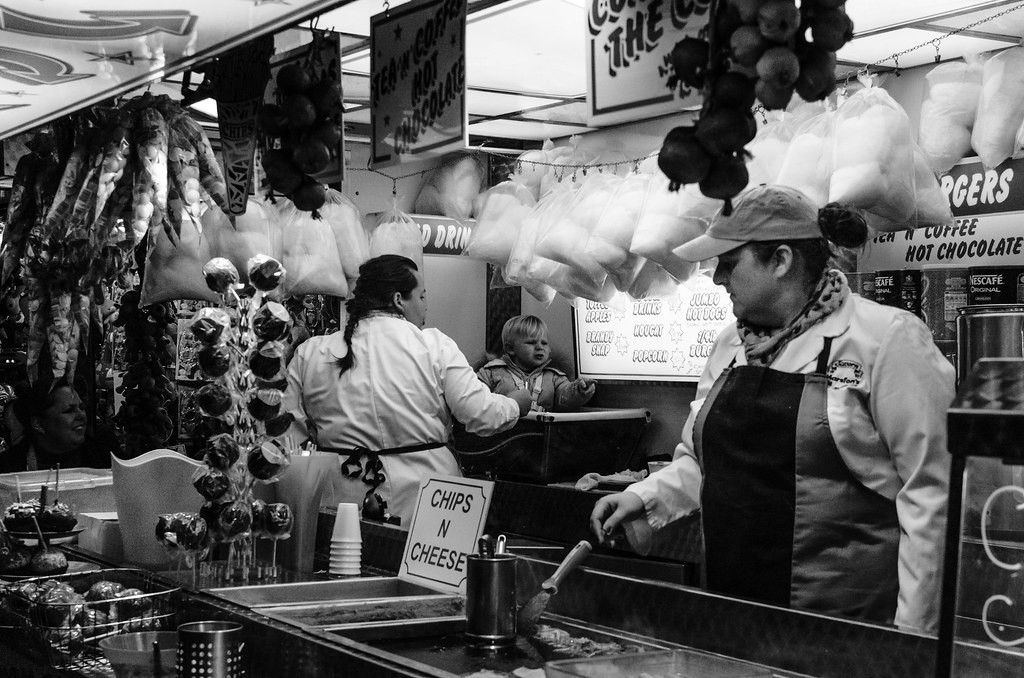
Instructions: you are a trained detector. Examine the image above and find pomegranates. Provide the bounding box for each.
[655,0,855,217]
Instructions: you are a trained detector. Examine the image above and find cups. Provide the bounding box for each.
[328,502,364,575]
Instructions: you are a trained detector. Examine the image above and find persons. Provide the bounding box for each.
[591,182,957,637]
[278,254,534,532]
[0,363,113,473]
[475,314,598,412]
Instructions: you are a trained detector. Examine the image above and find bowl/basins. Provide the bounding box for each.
[100,627,182,677]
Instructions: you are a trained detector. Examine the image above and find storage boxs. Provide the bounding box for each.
[78,511,123,559]
[0,467,118,537]
[452,407,652,486]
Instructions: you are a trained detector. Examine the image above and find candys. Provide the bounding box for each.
[0,460,155,665]
[154,255,296,586]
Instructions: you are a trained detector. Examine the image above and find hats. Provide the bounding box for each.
[672,184,823,263]
